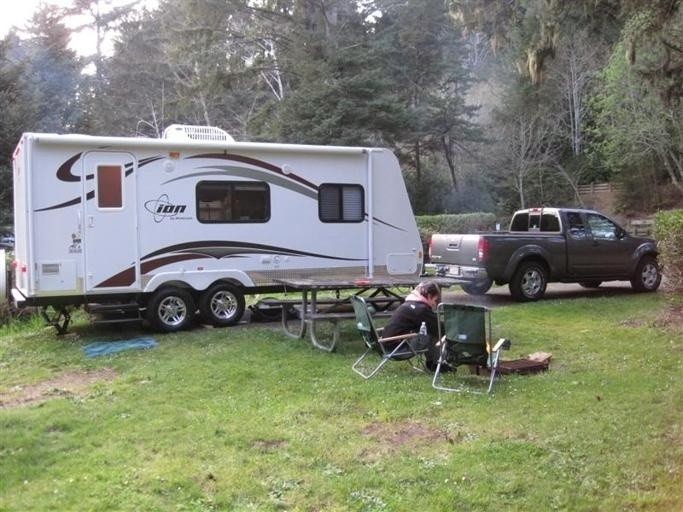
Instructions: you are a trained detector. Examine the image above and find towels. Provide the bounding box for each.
[81,337,158,357]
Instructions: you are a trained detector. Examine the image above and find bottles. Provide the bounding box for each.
[419,321,427,335]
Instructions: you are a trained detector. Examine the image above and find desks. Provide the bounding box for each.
[270,274,472,336]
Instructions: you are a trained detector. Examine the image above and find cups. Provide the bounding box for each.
[495,222,500,231]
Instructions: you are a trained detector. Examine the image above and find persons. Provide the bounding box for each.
[381,281,458,373]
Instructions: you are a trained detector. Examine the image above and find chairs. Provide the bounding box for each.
[429,299,511,399]
[347,291,440,382]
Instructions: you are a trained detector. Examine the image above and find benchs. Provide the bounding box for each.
[301,312,398,355]
[261,295,405,334]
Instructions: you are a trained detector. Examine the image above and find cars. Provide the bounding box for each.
[0,230,14,249]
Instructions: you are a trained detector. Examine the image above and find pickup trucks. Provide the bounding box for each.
[427,208,662,302]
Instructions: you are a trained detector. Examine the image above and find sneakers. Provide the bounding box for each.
[425,360,457,373]
